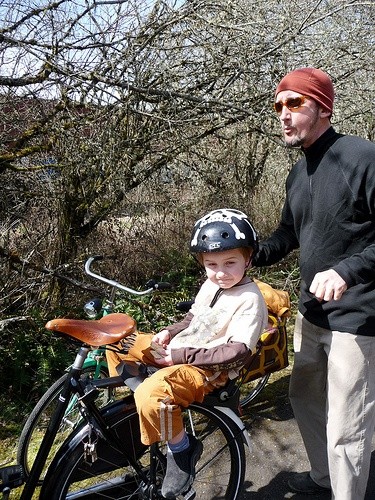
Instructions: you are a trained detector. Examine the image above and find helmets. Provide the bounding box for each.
[190,208,257,253]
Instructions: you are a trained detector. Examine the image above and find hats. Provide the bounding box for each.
[274,68,334,111]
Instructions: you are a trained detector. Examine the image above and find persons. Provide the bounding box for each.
[106,209,268,499]
[240,68,375,500]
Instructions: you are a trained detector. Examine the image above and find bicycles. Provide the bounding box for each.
[17,255,271,487]
[1,313,256,500]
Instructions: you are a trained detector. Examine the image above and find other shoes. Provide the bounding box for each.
[160,434,204,498]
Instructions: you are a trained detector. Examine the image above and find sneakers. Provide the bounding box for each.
[288,473,330,493]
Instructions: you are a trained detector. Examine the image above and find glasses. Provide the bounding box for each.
[275,96,308,113]
